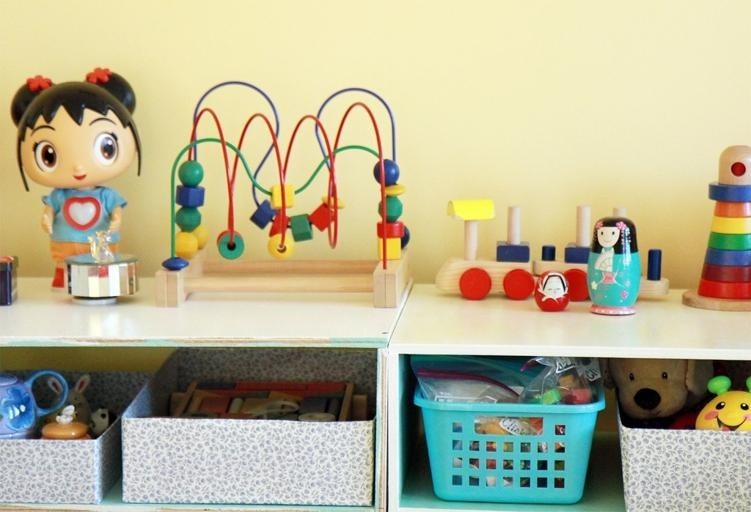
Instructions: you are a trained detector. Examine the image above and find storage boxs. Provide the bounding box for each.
[120,349,374,504]
[0,371,146,504]
[613,395,751,512]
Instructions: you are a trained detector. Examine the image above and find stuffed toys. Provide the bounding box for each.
[467,354,751,488]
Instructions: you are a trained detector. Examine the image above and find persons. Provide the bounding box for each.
[10,68,140,292]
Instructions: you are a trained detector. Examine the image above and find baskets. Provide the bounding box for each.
[413,385,605,506]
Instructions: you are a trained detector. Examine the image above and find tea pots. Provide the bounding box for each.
[0,369,69,438]
